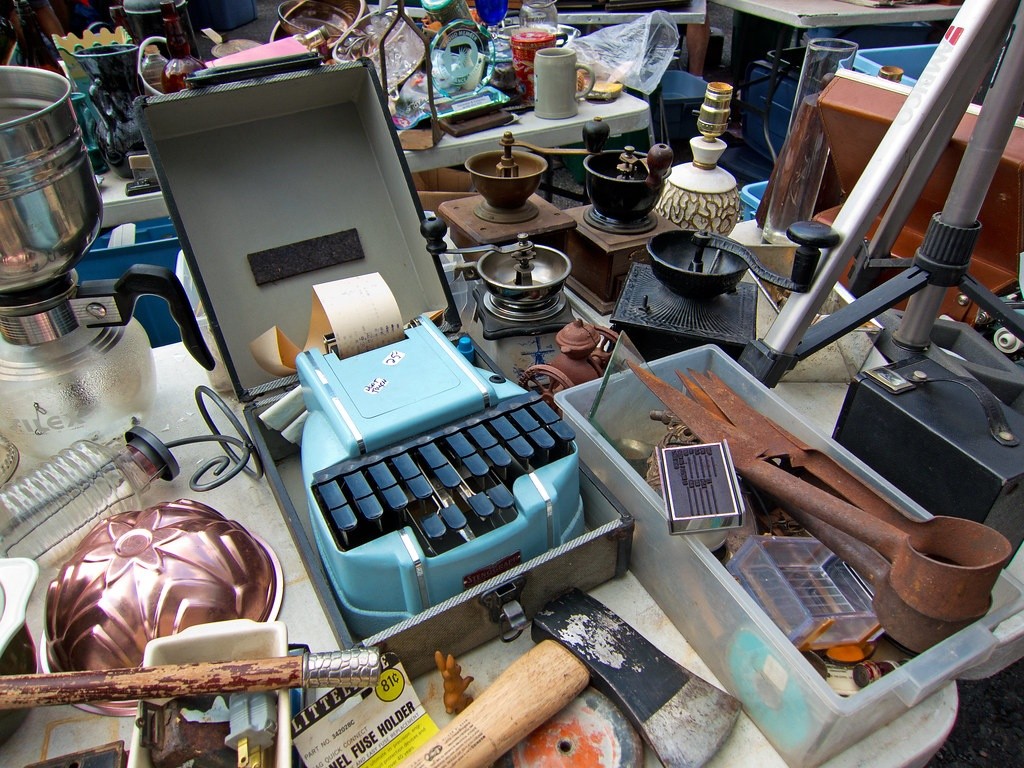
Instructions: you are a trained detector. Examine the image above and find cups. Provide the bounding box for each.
[761,37,859,244]
[513,32,567,106]
[533,48,595,119]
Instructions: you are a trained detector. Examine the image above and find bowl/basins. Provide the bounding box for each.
[331,6,425,91]
[498,24,581,48]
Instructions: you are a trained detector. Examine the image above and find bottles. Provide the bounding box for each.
[71,44,144,179]
[520,0,558,33]
[141,44,169,94]
[292,25,331,52]
[109,6,142,47]
[160,0,208,95]
[0,425,180,598]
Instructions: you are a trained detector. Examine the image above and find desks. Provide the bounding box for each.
[101,84,648,234]
[710,0,966,166]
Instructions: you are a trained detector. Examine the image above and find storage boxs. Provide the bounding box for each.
[129,56,636,674]
[628,66,709,138]
[553,343,1024,766]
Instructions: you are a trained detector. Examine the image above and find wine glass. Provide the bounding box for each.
[475,0,510,52]
